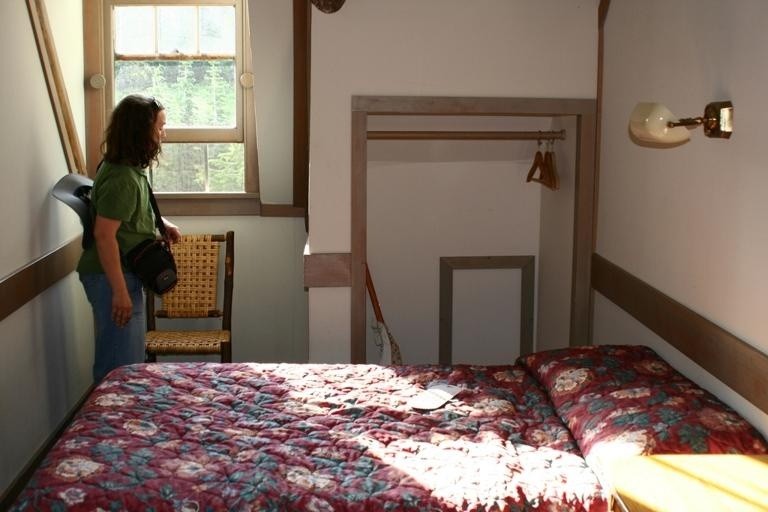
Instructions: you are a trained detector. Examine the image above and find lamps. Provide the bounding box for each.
[630,102,734,144]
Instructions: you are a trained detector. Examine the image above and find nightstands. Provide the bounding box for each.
[612,454,768,512]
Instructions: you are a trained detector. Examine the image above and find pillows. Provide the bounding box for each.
[522,345,768,454]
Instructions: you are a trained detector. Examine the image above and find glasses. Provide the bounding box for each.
[152,100,163,123]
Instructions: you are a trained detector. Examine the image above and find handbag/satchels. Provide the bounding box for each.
[126,239,178,295]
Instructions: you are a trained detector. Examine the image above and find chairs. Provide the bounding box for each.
[143,231,234,362]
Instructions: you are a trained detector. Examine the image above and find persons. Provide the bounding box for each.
[77,94,181,383]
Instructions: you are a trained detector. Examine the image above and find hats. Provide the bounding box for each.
[52,173,95,249]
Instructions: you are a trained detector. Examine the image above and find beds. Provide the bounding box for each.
[25,363,619,512]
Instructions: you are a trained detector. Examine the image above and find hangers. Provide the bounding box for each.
[526,129,560,190]
